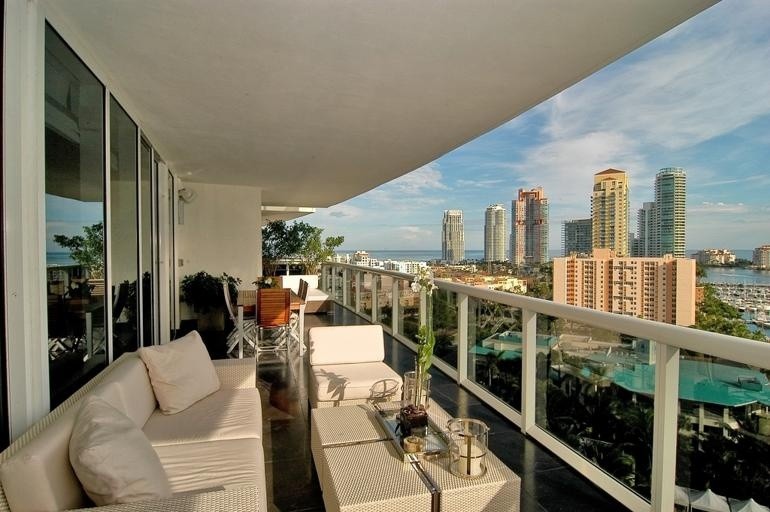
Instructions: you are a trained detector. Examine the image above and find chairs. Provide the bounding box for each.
[271,278,305,345]
[273,281,309,350]
[91,280,131,354]
[222,278,260,354]
[256,288,291,356]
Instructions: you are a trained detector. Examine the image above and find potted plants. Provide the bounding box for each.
[179,270,243,331]
[406,326,435,409]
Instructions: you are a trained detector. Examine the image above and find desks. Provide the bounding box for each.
[236,290,306,359]
[54,289,105,361]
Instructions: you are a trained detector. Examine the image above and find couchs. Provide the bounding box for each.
[281,275,332,314]
[0,353,266,512]
[308,324,405,406]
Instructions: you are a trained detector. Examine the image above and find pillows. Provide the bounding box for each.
[139,330,220,414]
[69,395,173,503]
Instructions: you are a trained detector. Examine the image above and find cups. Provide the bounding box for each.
[400,372,432,411]
[445,418,490,479]
